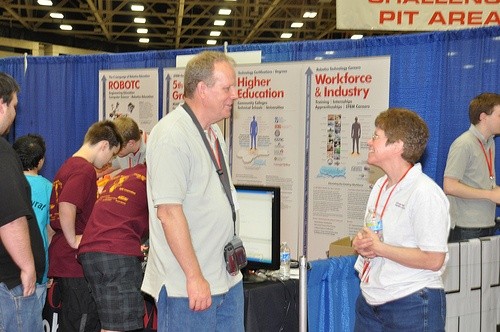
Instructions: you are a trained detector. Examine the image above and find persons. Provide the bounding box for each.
[77,162,149,332]
[443,92,500,243]
[48,119,123,332]
[113,116,149,168]
[16,134,55,312]
[0,71,45,332]
[352,108,451,332]
[141,51,245,332]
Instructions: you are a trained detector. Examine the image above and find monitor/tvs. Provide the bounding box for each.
[234,184,281,276]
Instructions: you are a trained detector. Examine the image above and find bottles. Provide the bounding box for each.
[280,242,291,280]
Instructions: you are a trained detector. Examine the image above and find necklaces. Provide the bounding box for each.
[129,133,147,169]
[477,137,494,180]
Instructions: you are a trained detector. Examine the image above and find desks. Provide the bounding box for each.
[41,279,299,332]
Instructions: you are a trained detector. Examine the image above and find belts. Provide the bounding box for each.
[455,225,497,231]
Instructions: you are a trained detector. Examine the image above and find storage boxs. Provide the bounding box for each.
[329,237,356,257]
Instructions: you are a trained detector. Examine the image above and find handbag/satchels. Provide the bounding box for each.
[47,281,62,312]
[142,300,157,331]
